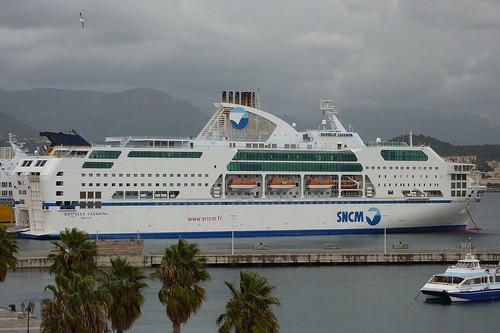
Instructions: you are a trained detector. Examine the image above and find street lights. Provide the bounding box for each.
[383,215,387,253]
[24,301,35,333]
[230,213,236,255]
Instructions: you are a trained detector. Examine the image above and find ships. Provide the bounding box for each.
[11,84,489,240]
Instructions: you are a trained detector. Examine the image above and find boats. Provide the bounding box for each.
[420,238,500,303]
[0,131,28,223]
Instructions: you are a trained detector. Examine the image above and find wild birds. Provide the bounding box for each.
[79,12,85,29]
